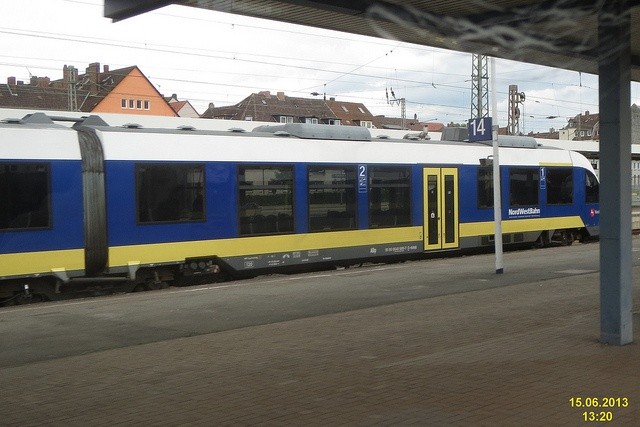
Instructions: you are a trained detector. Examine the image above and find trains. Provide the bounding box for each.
[0,114,601,302]
